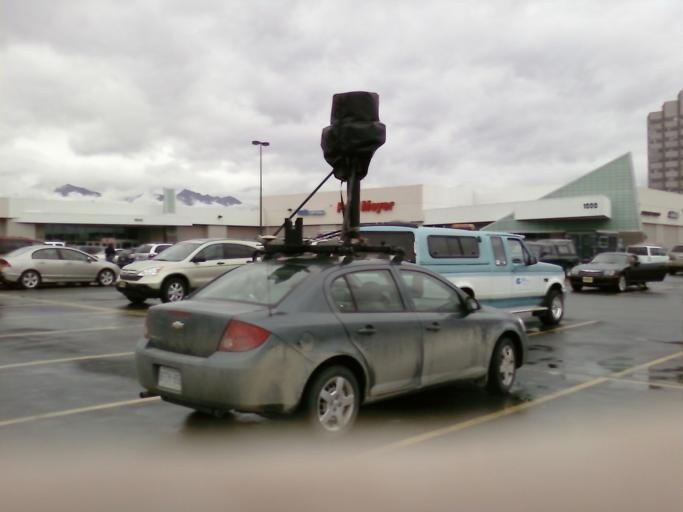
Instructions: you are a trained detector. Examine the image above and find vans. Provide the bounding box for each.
[358,225,564,328]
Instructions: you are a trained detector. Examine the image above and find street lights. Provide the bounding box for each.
[254,140,270,234]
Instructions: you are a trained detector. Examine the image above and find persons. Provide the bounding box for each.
[628,255,649,290]
[104,240,118,264]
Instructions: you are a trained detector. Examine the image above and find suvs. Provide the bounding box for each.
[116,239,263,302]
[535,241,578,272]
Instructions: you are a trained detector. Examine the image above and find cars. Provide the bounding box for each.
[569,243,683,294]
[0,245,119,290]
[138,261,529,439]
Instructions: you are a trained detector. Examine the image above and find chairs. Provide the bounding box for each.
[331,281,392,312]
